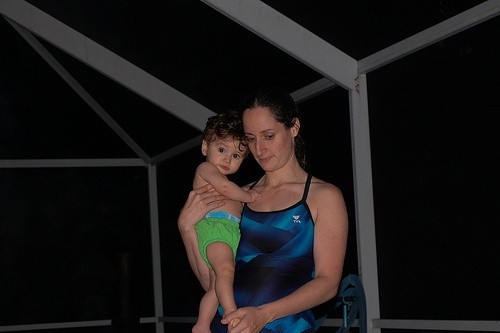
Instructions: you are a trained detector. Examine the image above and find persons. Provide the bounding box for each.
[177,85,349,332]
[192,111,262,332]
